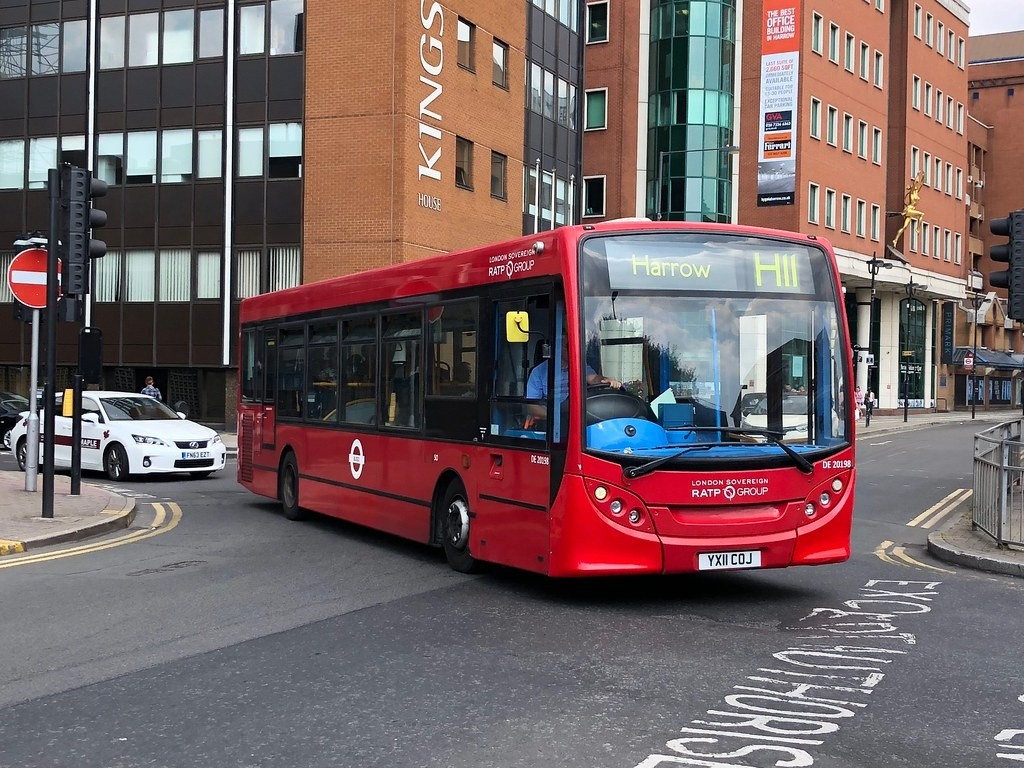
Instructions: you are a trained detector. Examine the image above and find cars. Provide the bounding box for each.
[740,395,839,445]
[0,391,29,449]
[10,391,227,481]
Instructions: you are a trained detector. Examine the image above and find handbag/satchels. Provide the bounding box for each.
[854,408,860,421]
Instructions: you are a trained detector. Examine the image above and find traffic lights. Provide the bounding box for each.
[990,210,1024,325]
[60,164,108,295]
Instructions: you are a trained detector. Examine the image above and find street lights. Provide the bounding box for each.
[904,276,919,422]
[970,292,986,419]
[866,251,884,427]
[14,238,62,491]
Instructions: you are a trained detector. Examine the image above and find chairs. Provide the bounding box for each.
[391,361,406,378]
[435,360,451,385]
[347,353,364,363]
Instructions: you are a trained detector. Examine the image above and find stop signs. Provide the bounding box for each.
[7,247,63,308]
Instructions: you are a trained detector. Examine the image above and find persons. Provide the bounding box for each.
[785,382,876,441]
[141,376,163,418]
[453,361,473,384]
[892,169,928,248]
[306,344,420,409]
[526,331,622,432]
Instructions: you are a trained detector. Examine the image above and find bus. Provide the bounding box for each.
[236,218,853,577]
